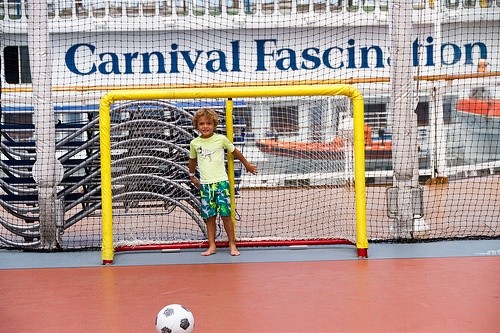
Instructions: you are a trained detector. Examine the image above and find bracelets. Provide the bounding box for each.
[189,172,195,176]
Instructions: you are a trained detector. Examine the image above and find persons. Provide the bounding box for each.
[189,109,258,256]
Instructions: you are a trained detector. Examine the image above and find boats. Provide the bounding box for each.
[254,115,456,175]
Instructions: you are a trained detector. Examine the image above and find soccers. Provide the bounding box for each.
[155,304,194,333]
